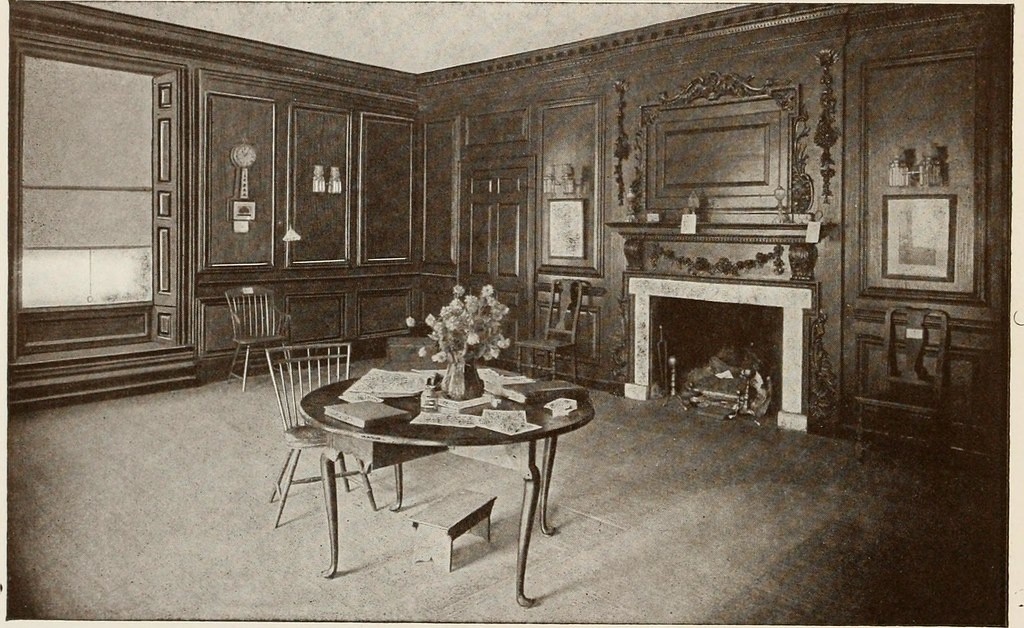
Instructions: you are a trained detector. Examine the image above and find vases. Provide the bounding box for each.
[441,351,485,399]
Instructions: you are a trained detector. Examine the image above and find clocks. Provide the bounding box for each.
[230,143,257,169]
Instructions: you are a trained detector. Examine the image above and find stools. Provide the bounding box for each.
[406,488,499,574]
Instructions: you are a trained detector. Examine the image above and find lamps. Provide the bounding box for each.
[311,165,342,194]
[887,140,941,188]
[542,162,574,196]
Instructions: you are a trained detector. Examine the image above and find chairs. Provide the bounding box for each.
[852,304,952,474]
[262,340,378,527]
[223,287,294,393]
[516,279,583,383]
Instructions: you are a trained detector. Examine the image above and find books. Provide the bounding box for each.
[324,401,411,429]
[502,380,590,404]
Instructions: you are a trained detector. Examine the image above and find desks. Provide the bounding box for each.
[297,366,595,609]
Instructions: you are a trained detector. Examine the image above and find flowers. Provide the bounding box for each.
[405,284,512,363]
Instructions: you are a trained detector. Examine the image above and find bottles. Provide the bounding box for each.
[543,164,555,194]
[313,165,325,193]
[921,141,943,187]
[889,143,908,187]
[420,385,439,409]
[562,164,574,194]
[328,166,342,194]
